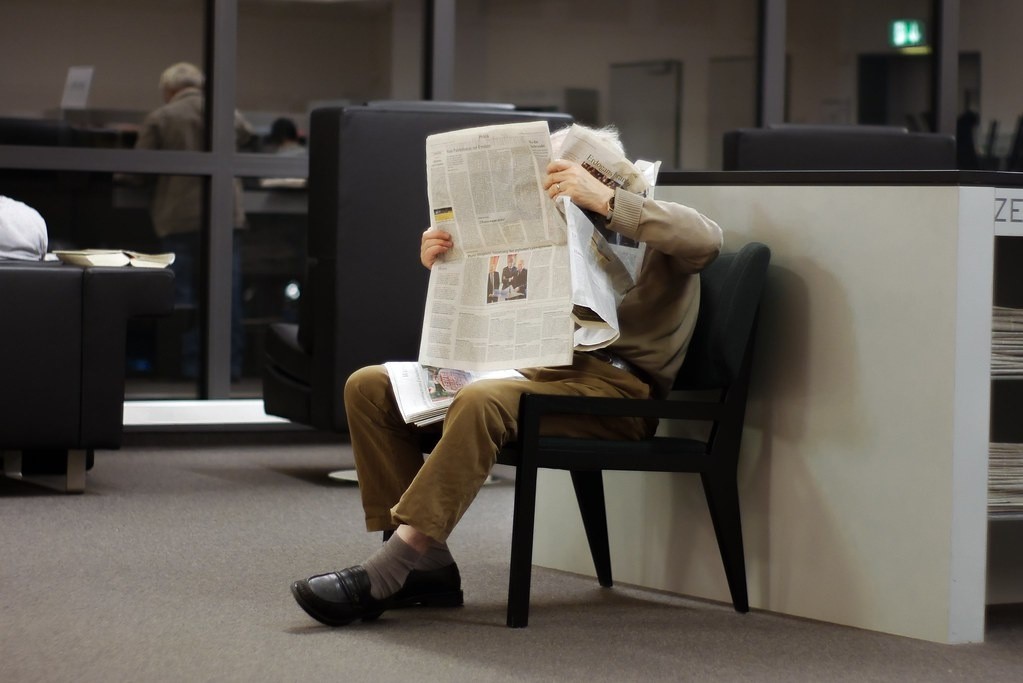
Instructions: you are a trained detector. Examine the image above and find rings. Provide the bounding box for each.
[556,183,561,192]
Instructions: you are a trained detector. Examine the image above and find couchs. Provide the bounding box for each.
[262,108,576,480]
[1,118,142,255]
[0,261,180,492]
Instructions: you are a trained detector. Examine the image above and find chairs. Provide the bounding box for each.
[419,242,770,632]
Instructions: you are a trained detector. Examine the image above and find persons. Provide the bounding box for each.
[501,257,527,301]
[132,64,296,400]
[487,264,500,303]
[582,161,615,189]
[290,122,723,626]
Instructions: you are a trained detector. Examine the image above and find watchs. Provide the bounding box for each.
[607,191,615,219]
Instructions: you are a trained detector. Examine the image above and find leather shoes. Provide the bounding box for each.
[386,562,465,608]
[289,565,423,627]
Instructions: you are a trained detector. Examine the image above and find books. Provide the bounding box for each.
[52,249,175,269]
